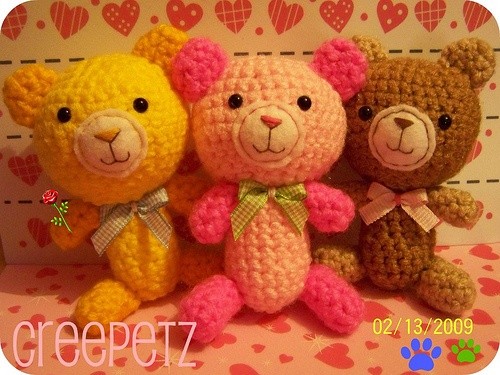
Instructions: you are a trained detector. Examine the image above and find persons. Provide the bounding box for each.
[171,37,369,344]
[3,23,225,337]
[311,35,496,315]
